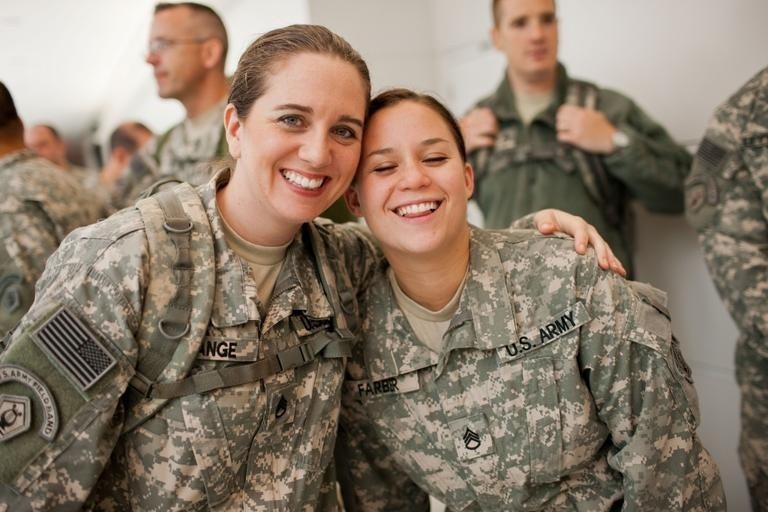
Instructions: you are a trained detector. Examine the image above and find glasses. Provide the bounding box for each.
[147,33,205,53]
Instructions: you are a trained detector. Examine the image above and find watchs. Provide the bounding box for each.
[603,131,631,157]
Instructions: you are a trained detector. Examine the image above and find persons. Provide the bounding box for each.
[0,81,94,340]
[96,2,230,219]
[334,89,726,512]
[0,25,628,512]
[685,67,768,512]
[80,122,154,200]
[457,0,692,282]
[24,125,88,183]
